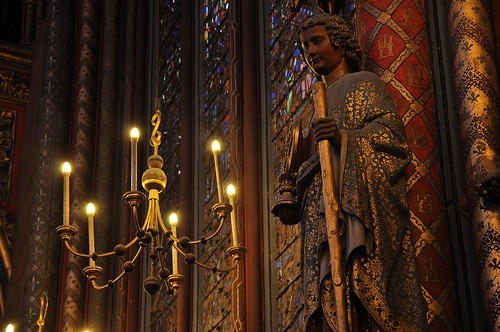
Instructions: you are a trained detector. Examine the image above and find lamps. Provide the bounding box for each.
[57,0,247,305]
